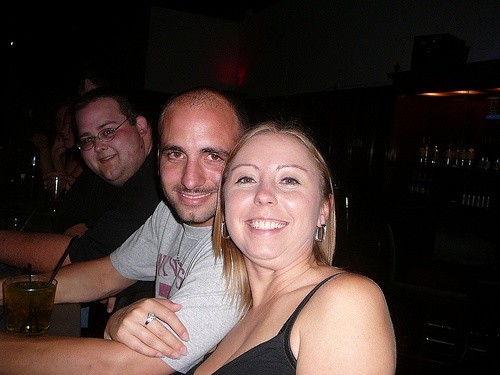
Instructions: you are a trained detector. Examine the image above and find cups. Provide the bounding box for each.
[3,276,58,335]
[46,177,66,198]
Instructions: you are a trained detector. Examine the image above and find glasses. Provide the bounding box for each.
[74,113,138,150]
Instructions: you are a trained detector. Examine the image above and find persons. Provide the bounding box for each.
[2,84,166,341]
[30,98,87,201]
[103,120,397,375]
[1,89,253,375]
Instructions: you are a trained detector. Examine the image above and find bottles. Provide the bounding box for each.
[408,133,500,210]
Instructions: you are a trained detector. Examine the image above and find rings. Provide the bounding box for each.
[144,312,155,326]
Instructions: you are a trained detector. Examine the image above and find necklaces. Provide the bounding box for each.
[176,217,219,267]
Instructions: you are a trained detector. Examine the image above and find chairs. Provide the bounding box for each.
[386,206,485,375]
